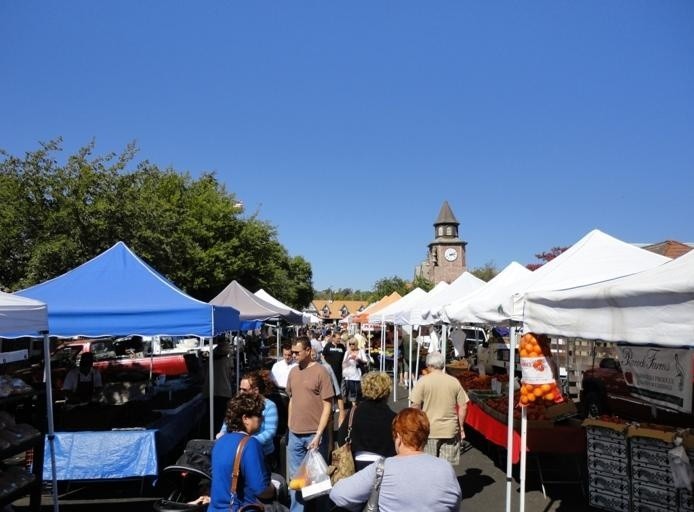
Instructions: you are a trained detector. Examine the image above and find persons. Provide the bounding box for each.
[319,331,346,388]
[328,408,462,512]
[336,372,398,472]
[286,335,335,510]
[216,373,279,463]
[439,331,455,363]
[406,351,469,467]
[310,338,347,433]
[208,391,276,512]
[63,351,101,409]
[403,331,421,380]
[271,342,299,395]
[342,336,368,403]
[203,339,232,424]
[375,323,402,348]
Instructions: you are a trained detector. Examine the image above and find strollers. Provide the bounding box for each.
[151,439,221,512]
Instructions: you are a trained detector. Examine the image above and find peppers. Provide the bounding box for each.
[472,389,496,396]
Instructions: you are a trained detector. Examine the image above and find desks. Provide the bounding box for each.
[456,391,587,499]
[31,391,210,486]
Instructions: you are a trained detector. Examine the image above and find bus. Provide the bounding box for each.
[485,345,575,387]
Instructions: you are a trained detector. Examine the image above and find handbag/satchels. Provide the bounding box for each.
[230,436,268,512]
[331,405,355,486]
[363,458,385,512]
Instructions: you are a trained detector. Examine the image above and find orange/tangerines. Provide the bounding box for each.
[519,333,565,406]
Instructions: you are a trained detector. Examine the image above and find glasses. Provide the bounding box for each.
[239,388,252,392]
[349,343,356,346]
[291,350,301,357]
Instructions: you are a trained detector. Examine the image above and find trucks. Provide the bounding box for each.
[581,342,694,423]
[14,340,204,391]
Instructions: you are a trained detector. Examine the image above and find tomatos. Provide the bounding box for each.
[485,394,521,419]
[640,423,674,434]
[527,402,548,421]
[597,414,626,425]
[494,373,510,383]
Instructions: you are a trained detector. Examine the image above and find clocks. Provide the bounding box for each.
[443,248,458,262]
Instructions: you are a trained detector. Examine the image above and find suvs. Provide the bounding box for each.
[439,324,487,361]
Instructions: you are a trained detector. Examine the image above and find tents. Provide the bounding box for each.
[470,227,673,512]
[368,260,535,407]
[519,248,694,511]
[254,288,323,350]
[1,290,58,512]
[207,280,305,392]
[337,291,403,372]
[15,240,239,442]
